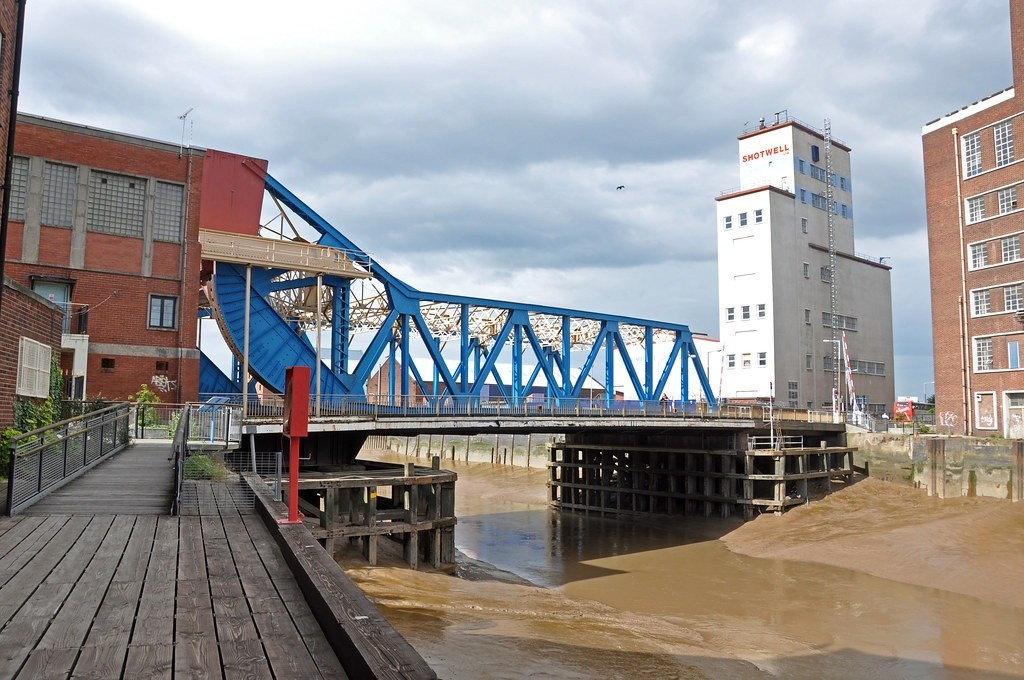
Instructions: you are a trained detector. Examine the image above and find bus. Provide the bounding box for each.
[893,401,935,423]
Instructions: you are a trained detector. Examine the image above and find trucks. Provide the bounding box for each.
[523,387,625,402]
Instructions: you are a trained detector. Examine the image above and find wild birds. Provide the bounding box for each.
[616,185,625,190]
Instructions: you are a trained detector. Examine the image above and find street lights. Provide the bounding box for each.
[706,348,722,384]
[923,382,935,404]
[769,380,775,450]
[589,364,594,416]
[822,337,842,416]
